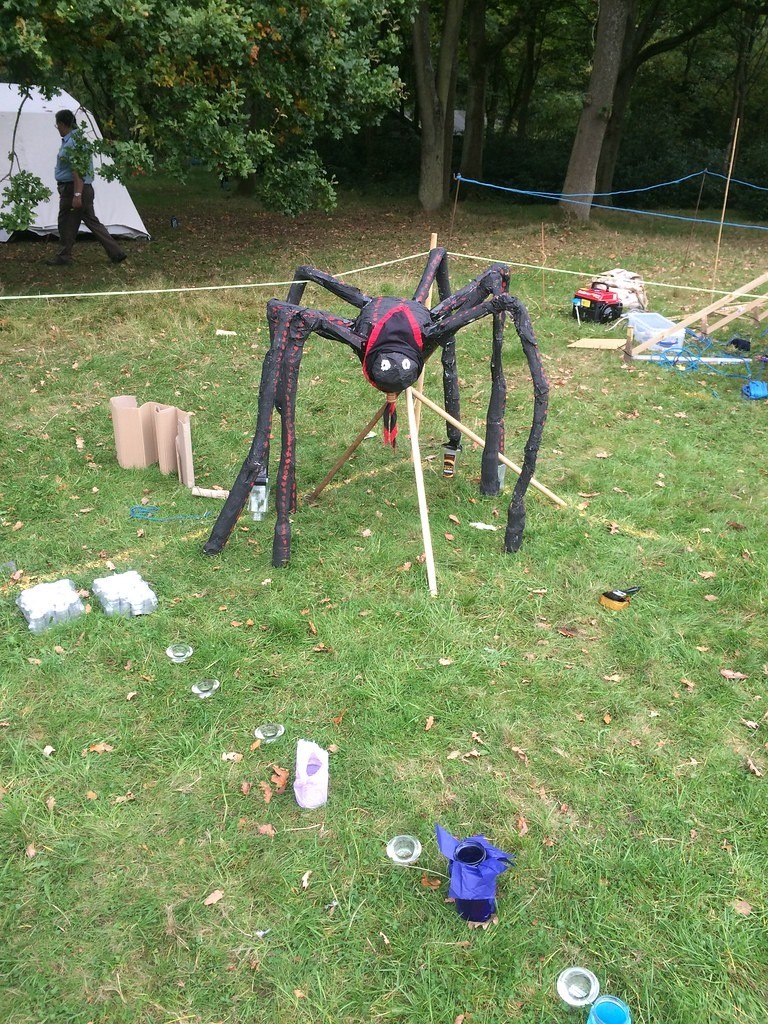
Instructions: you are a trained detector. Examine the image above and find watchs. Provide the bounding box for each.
[74,192,81,197]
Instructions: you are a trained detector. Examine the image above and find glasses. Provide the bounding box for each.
[54,124,59,129]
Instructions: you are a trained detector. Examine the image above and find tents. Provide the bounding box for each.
[0,83,150,243]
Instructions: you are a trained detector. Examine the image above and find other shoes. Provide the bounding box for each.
[111,252,127,264]
[45,254,73,265]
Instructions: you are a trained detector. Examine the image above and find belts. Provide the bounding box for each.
[57,182,64,185]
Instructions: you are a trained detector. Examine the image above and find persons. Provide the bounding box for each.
[45,109,128,267]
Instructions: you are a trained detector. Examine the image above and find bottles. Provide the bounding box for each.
[447,839,496,923]
[587,995,634,1024]
